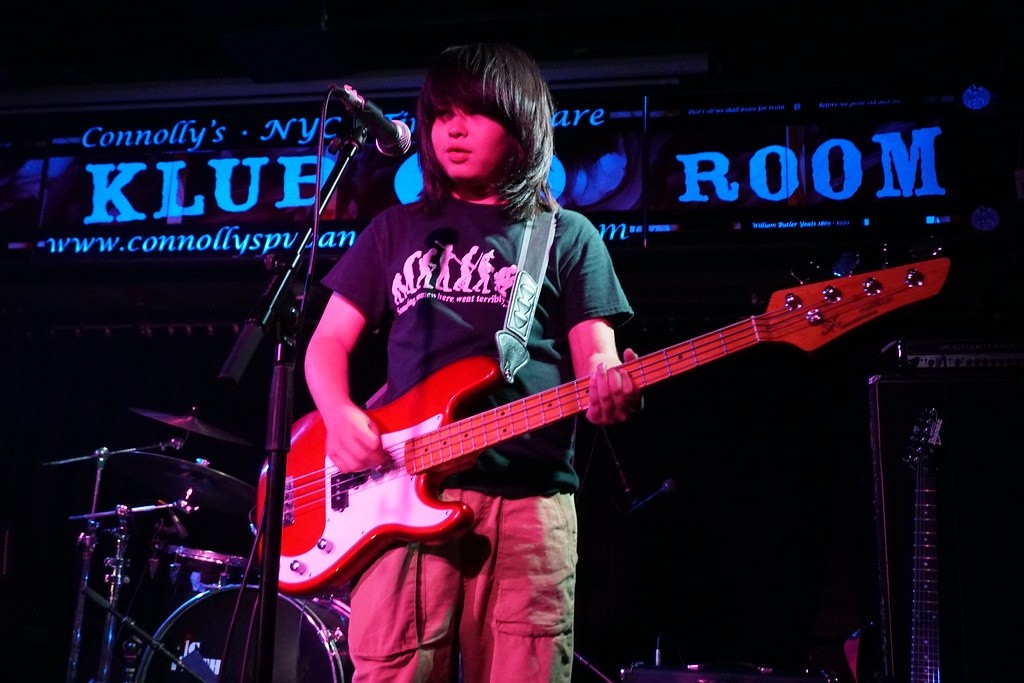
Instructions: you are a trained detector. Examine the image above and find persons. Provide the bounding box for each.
[303,41,651,683]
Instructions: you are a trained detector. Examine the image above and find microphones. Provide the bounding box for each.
[329,83,412,156]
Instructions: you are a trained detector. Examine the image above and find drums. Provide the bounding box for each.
[131,582,355,683]
[154,544,248,599]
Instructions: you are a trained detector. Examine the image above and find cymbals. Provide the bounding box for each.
[108,450,257,517]
[128,405,254,448]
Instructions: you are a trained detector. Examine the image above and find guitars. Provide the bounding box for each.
[254,235,953,597]
[898,401,946,683]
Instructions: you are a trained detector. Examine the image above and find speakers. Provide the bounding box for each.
[869,372,1024,683]
